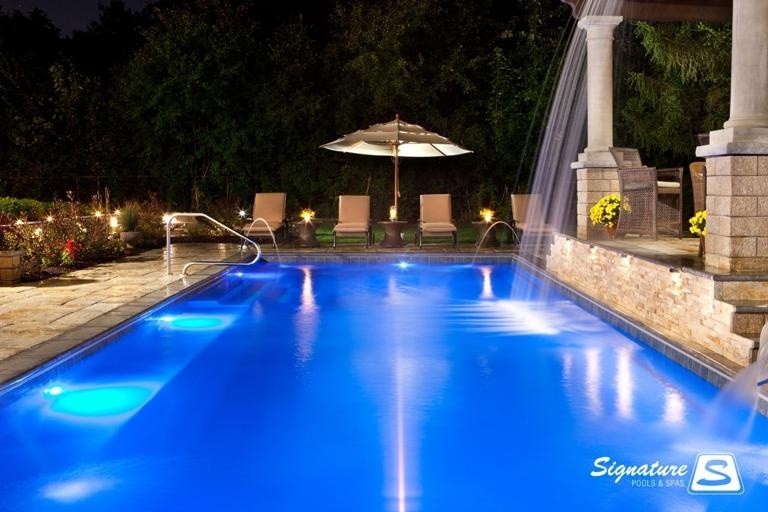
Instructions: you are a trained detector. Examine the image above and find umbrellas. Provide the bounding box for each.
[319,114,474,207]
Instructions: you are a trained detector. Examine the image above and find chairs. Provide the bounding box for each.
[333,195,372,248]
[243,192,286,248]
[416,194,458,248]
[507,194,559,246]
[609,147,684,240]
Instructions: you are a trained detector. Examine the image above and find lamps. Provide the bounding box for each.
[388,206,399,222]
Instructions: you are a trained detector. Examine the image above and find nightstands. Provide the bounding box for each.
[471,221,504,249]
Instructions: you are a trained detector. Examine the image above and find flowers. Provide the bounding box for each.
[3,216,81,277]
[688,210,707,235]
[590,195,630,227]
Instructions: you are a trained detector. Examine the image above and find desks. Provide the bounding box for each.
[377,221,409,248]
[291,221,324,248]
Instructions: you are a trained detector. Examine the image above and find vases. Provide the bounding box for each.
[699,238,704,257]
[0,248,25,284]
[607,225,625,240]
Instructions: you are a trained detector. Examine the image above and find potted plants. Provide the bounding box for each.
[119,201,143,254]
